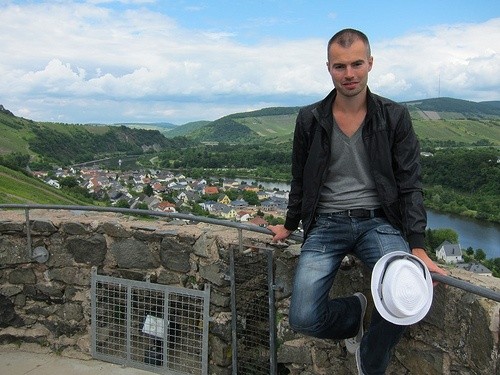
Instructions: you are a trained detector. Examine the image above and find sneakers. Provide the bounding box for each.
[345,292,367,355]
[355,346,365,375]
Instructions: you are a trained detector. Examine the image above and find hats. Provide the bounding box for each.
[370,251,433,326]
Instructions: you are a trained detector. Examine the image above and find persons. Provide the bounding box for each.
[267,29,449,375]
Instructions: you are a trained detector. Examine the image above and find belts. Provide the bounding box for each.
[319,208,386,219]
